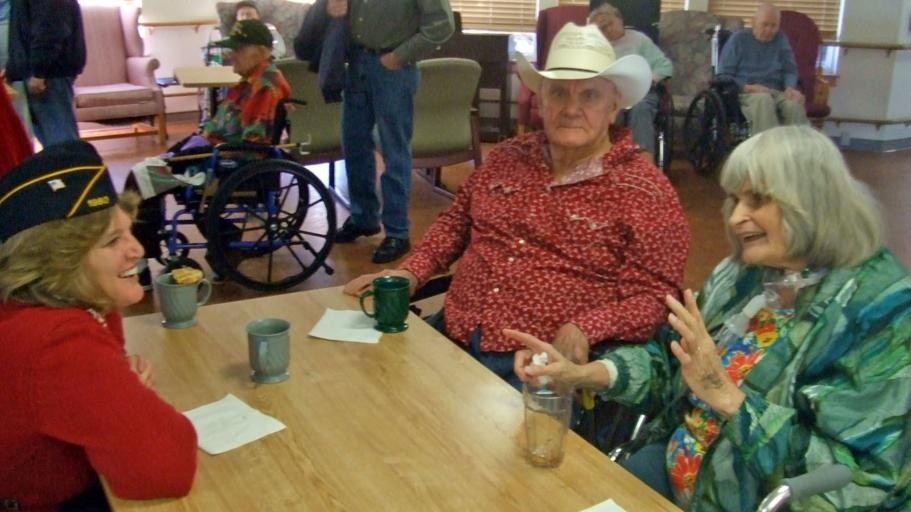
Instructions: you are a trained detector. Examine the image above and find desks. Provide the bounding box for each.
[90,283,681,511]
[428,32,515,144]
[174,64,244,122]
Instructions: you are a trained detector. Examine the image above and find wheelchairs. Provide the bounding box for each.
[650,83,676,170]
[683,28,752,180]
[127,97,335,291]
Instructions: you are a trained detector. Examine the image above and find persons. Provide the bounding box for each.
[502,126,911,510]
[588,5,674,164]
[716,3,807,134]
[341,22,691,398]
[308,0,455,265]
[236,1,286,61]
[2,141,197,512]
[125,19,292,290]
[5,0,88,147]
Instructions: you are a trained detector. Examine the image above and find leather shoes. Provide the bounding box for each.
[335,225,381,242]
[373,235,410,264]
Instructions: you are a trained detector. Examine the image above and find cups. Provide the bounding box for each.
[154,272,212,329]
[521,373,576,469]
[246,317,291,384]
[359,275,410,333]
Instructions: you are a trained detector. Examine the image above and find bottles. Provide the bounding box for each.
[209,26,223,68]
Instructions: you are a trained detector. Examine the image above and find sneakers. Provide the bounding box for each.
[138,266,152,291]
[212,228,243,285]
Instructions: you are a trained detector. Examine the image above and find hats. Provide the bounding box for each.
[514,22,653,110]
[215,19,273,47]
[0,139,118,243]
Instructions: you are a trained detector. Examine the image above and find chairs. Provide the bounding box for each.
[271,60,352,233]
[412,56,484,197]
[405,272,632,441]
[780,10,832,137]
[657,10,744,157]
[516,4,588,135]
[73,5,168,145]
[605,410,855,511]
